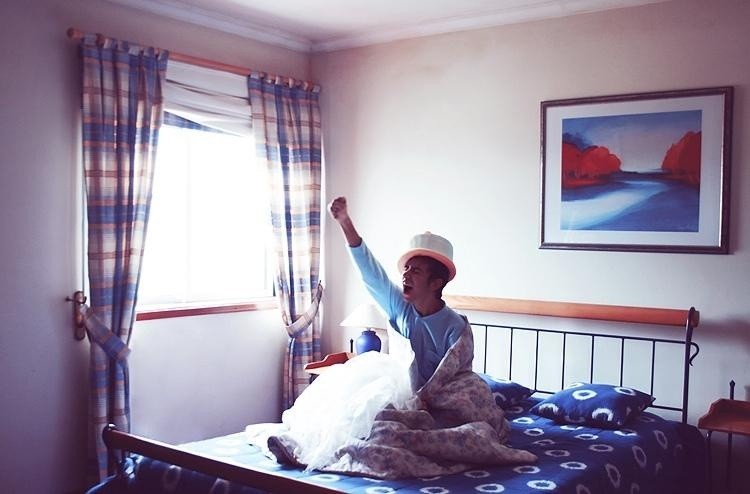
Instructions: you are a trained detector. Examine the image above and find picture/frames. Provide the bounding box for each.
[538,84,733,254]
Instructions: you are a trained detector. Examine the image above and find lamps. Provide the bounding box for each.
[339,303,390,355]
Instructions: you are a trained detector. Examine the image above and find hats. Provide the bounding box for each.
[397,229,456,283]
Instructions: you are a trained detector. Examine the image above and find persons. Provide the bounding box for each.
[327,195,467,382]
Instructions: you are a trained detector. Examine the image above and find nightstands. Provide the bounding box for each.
[304,339,356,383]
[699,378,750,494]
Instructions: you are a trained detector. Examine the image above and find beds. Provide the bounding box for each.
[102,295,700,492]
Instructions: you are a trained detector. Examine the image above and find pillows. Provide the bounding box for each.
[527,381,656,430]
[474,370,544,419]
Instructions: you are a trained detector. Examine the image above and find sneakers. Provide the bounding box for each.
[267,436,313,468]
